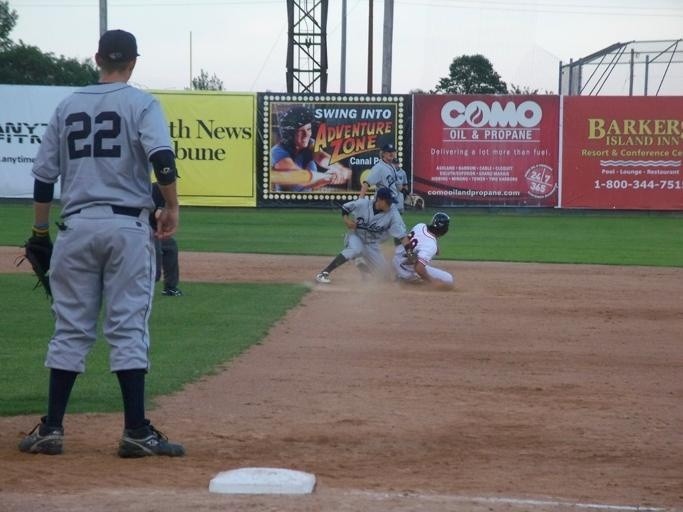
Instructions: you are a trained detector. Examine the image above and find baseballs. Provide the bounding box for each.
[359,170,378,191]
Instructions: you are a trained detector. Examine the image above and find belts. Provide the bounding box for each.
[73,205,141,218]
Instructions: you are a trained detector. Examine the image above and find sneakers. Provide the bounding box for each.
[19,415,63,455]
[360,272,367,279]
[161,286,183,297]
[118,420,186,458]
[316,272,331,283]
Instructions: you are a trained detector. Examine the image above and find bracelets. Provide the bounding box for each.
[31,227,48,234]
[33,222,48,228]
[402,243,412,248]
[404,249,414,255]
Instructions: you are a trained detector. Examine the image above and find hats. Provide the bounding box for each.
[96,30,141,61]
[393,158,400,164]
[384,144,395,152]
[378,188,398,204]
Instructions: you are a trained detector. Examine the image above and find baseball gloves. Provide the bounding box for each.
[401,245,417,265]
[15,232,53,300]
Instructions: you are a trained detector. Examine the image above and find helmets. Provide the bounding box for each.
[279,106,313,149]
[429,212,450,236]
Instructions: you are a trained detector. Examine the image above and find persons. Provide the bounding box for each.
[151,168,181,297]
[271,107,352,193]
[391,158,409,216]
[359,142,413,246]
[17,28,186,458]
[315,188,417,284]
[391,213,454,292]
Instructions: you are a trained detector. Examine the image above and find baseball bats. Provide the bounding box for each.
[271,170,334,186]
